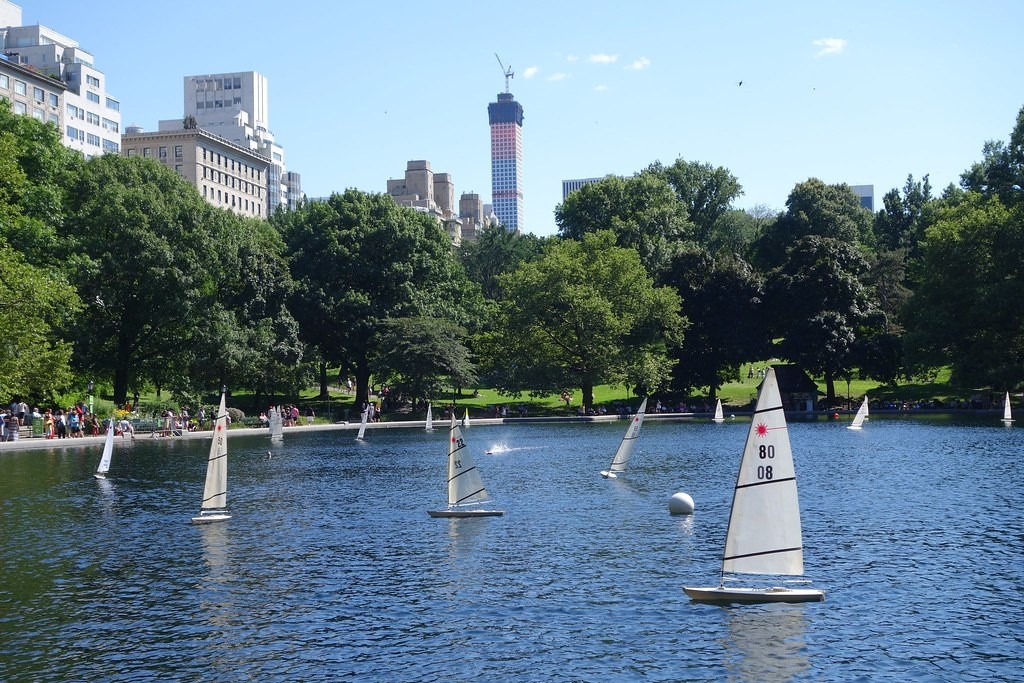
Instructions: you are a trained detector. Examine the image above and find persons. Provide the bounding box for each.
[577,397,718,416]
[338,376,391,423]
[817,389,1013,412]
[748,364,765,378]
[161,404,232,438]
[259,404,315,427]
[0,402,137,442]
[444,389,528,420]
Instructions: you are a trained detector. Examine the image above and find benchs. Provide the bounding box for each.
[241,416,266,428]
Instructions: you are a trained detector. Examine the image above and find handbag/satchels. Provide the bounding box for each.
[57,420,63,428]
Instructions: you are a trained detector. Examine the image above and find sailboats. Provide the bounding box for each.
[266,406,284,439]
[191,384,232,525]
[1001,390,1017,422]
[846,395,869,430]
[599,397,648,479]
[354,405,370,443]
[427,413,504,517]
[93,416,114,480]
[463,407,471,426]
[712,398,726,421]
[423,404,438,431]
[680,365,826,603]
